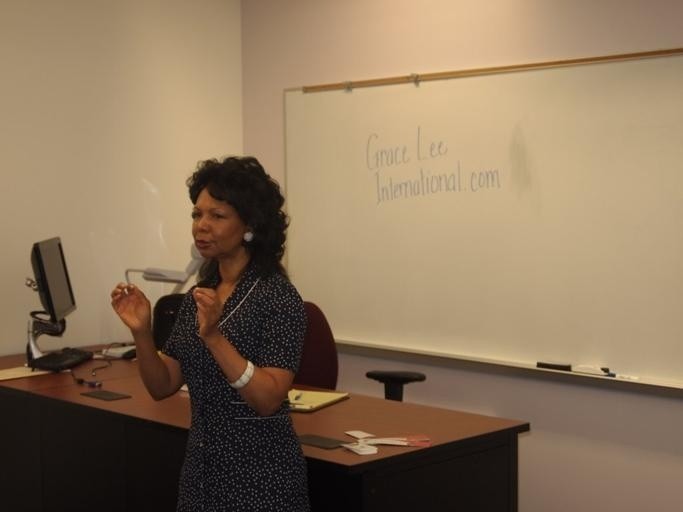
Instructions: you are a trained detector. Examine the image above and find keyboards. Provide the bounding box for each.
[34,348,90,371]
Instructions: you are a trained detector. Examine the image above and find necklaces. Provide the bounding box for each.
[194,276,261,338]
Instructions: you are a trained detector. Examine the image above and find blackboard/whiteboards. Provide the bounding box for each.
[283,48,683,391]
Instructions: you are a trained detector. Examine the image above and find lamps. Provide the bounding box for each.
[123,265,188,289]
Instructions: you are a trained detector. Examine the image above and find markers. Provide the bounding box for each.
[606,373,641,381]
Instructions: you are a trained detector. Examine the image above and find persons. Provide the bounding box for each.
[110,156,312,511]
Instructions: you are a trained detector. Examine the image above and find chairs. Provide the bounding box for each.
[292,302,426,401]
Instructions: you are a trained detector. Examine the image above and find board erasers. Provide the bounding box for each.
[535,362,573,371]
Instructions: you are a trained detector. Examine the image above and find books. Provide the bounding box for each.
[287,388,348,413]
[94,343,134,359]
[0,366,51,381]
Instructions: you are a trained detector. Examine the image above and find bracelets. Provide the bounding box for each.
[228,359,254,390]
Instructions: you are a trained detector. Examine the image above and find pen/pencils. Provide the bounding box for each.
[295,392,302,401]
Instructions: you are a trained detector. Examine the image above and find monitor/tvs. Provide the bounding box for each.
[30,236,76,323]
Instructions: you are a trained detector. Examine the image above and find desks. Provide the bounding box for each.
[0,344,530,511]
